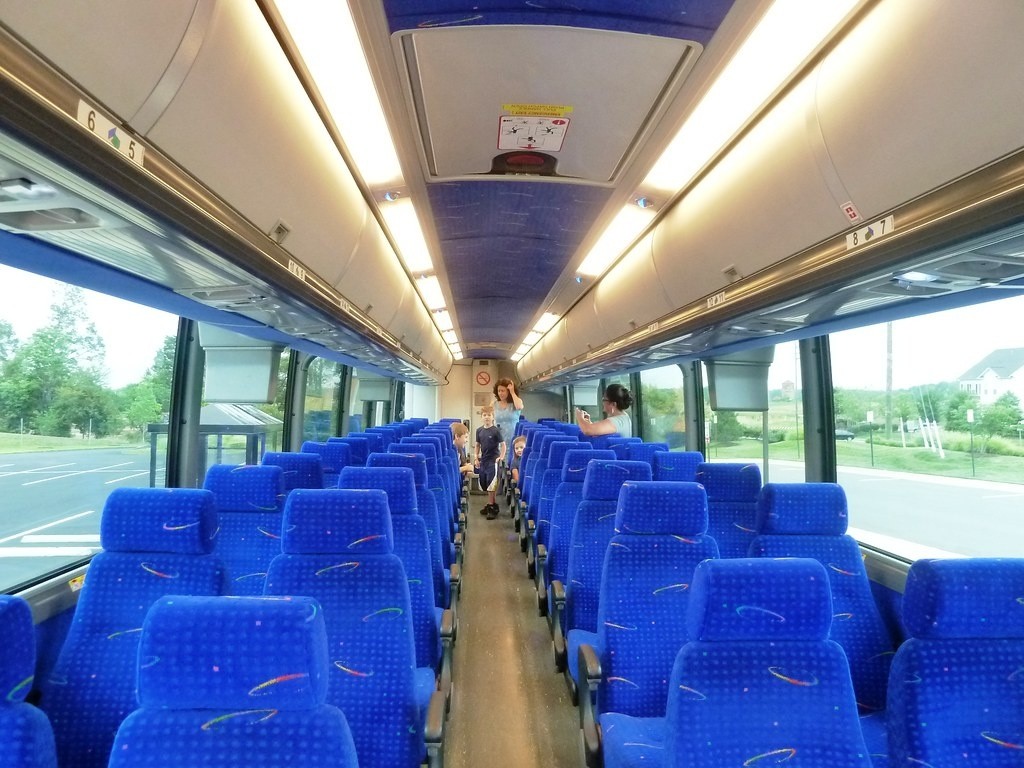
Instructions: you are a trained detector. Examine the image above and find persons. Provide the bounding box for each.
[450,422,473,475]
[489,378,527,495]
[475,407,506,520]
[576,384,634,438]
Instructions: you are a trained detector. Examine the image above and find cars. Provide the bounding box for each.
[835,430,855,441]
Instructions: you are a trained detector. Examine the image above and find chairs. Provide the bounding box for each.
[499,416,1024,768]
[0,417,473,768]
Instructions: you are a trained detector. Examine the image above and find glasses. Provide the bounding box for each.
[602,398,609,401]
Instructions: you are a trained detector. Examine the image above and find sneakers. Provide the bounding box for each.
[487,503,499,520]
[480,506,488,515]
